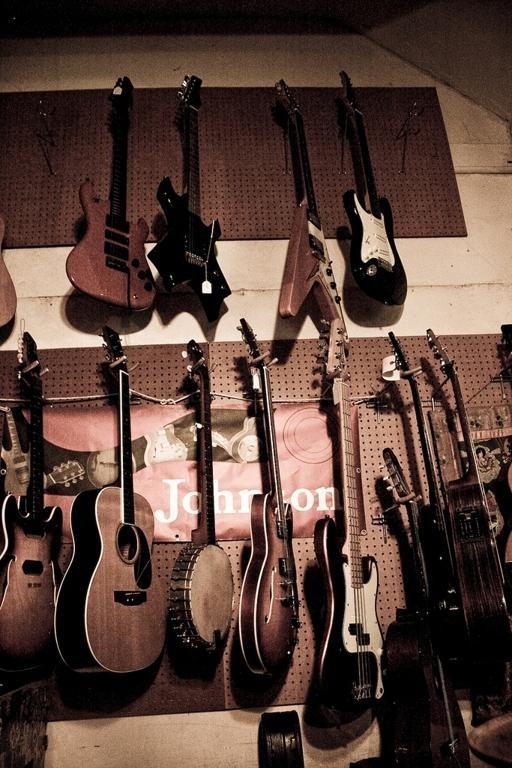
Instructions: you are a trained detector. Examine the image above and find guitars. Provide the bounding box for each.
[376,447,473,768]
[190,419,229,455]
[238,319,301,676]
[427,328,512,662]
[308,318,386,727]
[338,70,409,306]
[0,332,64,674]
[389,332,470,669]
[47,460,85,490]
[53,325,167,679]
[143,423,188,467]
[169,338,234,655]
[230,417,258,464]
[1,406,32,499]
[86,446,136,489]
[273,78,351,354]
[65,77,154,311]
[146,75,232,323]
[504,462,512,563]
[0,217,18,328]
